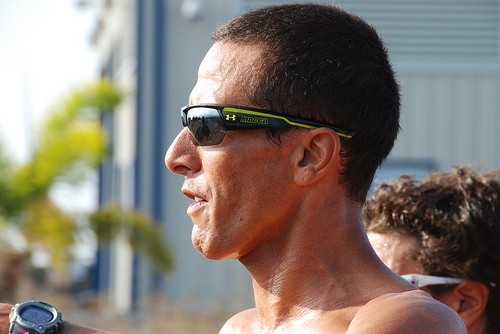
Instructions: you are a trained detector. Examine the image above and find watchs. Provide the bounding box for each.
[8,300,63,334]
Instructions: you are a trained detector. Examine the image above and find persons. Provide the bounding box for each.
[362,167,500,334]
[0,2,468,334]
